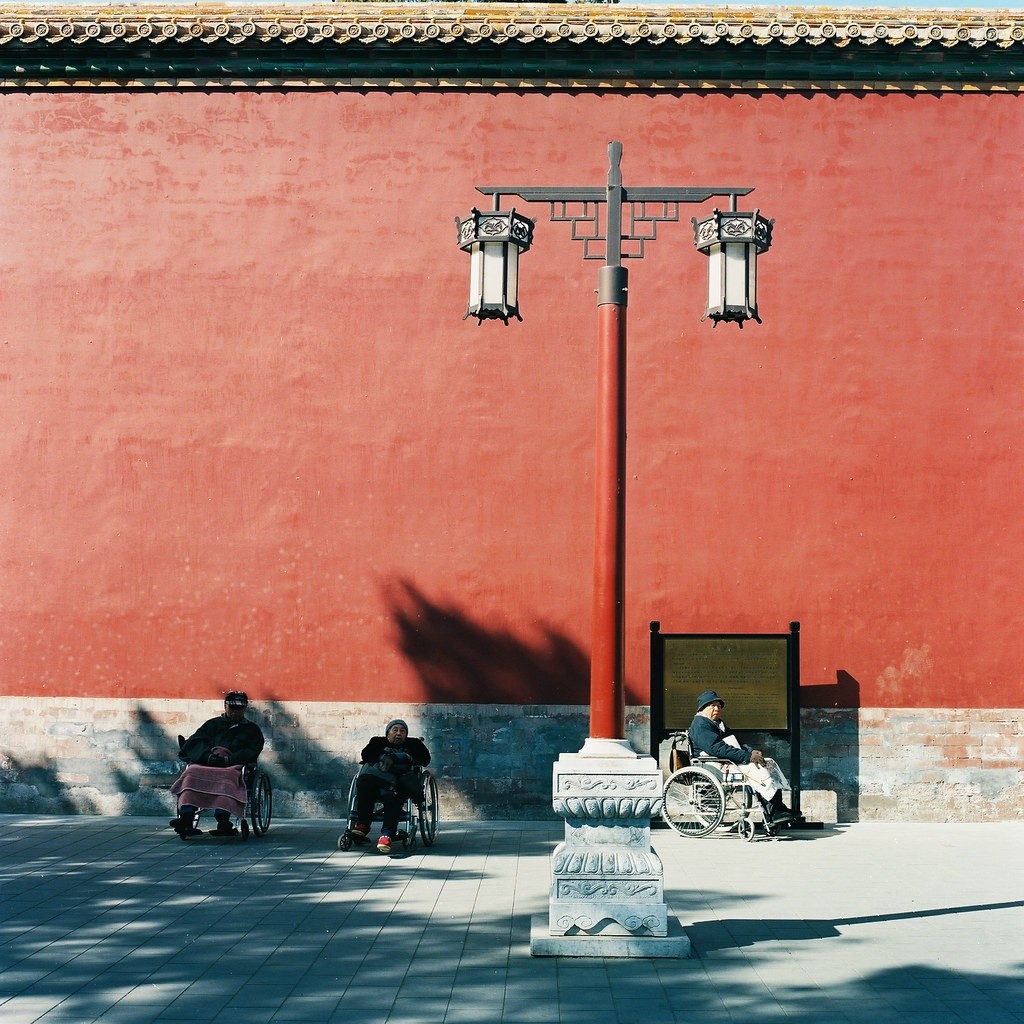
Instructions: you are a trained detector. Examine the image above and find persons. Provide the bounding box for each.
[690,690,804,824]
[351,718,430,846]
[170,690,266,833]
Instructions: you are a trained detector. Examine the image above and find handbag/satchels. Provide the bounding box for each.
[669,748,692,785]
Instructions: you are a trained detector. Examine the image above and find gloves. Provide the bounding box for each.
[751,750,762,757]
[750,754,767,769]
[381,755,394,772]
[209,754,225,767]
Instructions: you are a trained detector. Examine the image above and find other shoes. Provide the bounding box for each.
[217,818,233,830]
[783,807,802,818]
[169,814,194,831]
[377,835,393,854]
[351,821,371,837]
[772,810,792,823]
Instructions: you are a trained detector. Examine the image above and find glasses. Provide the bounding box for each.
[227,705,245,710]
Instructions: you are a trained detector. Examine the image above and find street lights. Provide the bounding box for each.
[452,137,775,958]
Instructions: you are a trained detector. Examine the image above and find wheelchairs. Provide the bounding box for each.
[177,762,272,839]
[659,731,790,842]
[337,761,438,850]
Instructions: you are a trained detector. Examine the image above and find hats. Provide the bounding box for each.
[224,691,248,706]
[385,720,408,736]
[696,691,724,712]
